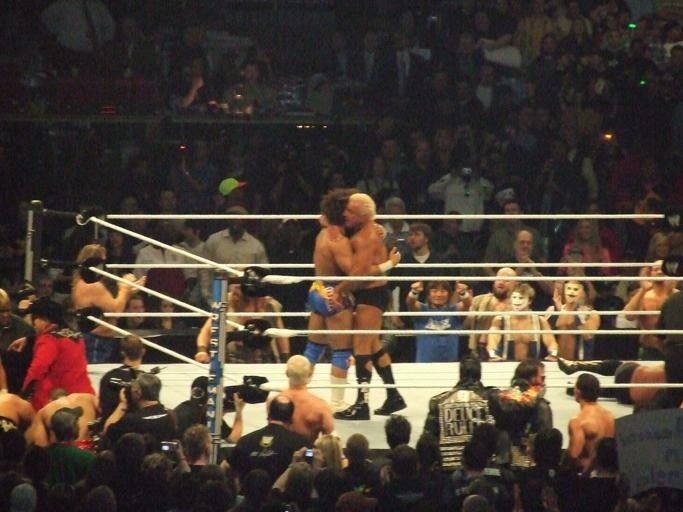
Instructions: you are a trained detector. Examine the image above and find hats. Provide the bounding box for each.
[19,296,61,317]
[219,178,246,196]
[192,377,208,402]
[53,407,82,426]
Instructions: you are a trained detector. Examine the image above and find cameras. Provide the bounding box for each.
[160,441,178,452]
[223,387,241,412]
[305,449,314,457]
[460,168,472,176]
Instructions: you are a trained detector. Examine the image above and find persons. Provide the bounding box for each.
[1,0,682,511]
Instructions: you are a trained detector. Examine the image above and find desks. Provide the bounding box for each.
[0,106,383,145]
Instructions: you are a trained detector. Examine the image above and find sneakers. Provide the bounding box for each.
[331,400,369,419]
[375,394,407,414]
